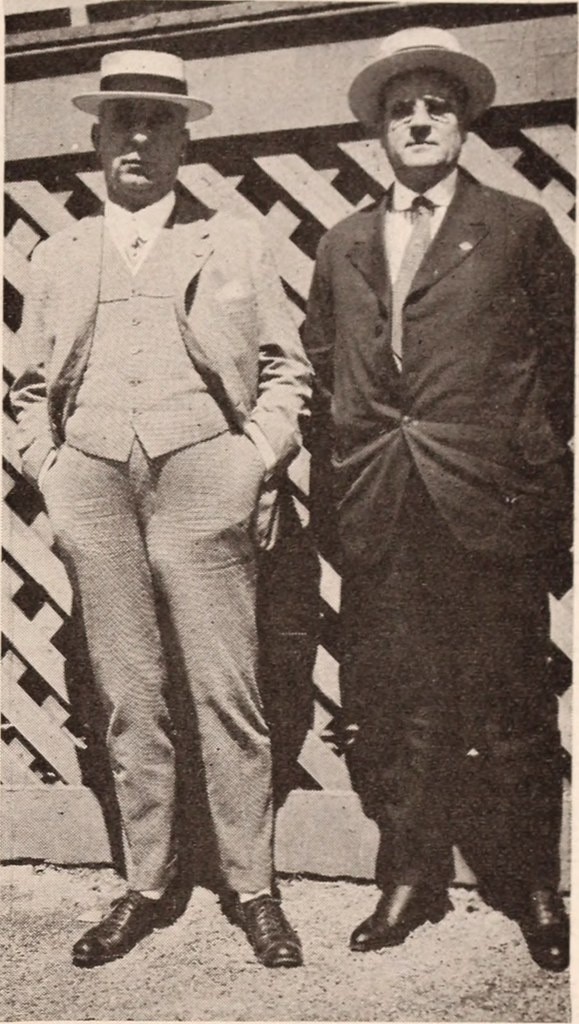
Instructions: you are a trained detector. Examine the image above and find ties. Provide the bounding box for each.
[391,196,431,360]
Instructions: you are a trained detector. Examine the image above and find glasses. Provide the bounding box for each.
[378,101,458,130]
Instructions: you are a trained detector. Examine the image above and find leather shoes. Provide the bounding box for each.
[352,885,448,950]
[517,889,569,972]
[237,895,302,963]
[71,881,179,959]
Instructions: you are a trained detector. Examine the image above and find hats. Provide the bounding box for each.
[72,50,214,122]
[348,27,496,129]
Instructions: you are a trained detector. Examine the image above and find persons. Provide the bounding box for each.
[9,48,315,967]
[297,26,572,974]
